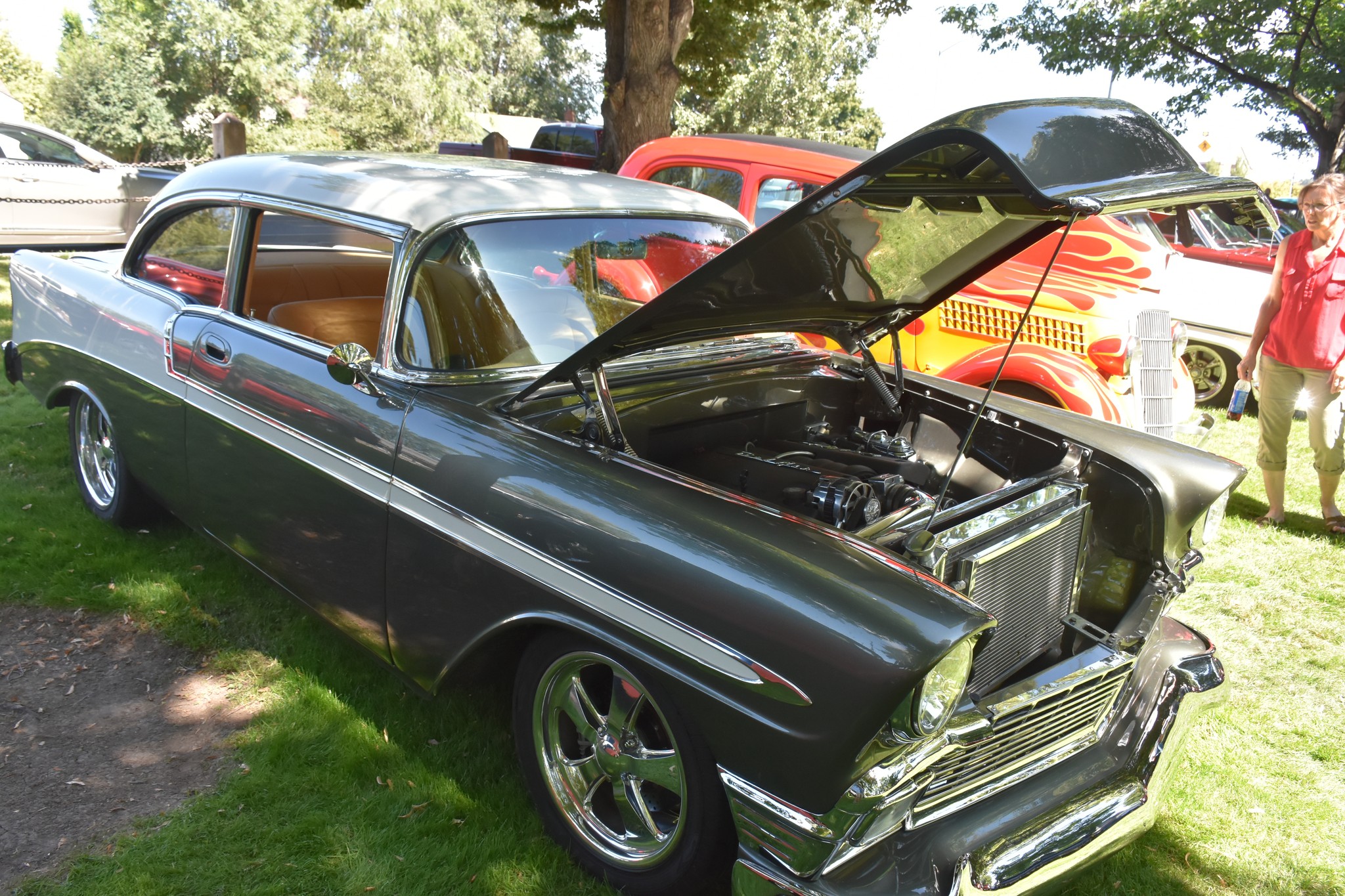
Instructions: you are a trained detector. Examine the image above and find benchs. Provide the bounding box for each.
[249,257,431,324]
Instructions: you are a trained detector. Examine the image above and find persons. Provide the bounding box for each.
[1236,173,1344,532]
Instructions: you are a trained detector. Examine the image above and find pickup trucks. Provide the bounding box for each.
[436,122,605,170]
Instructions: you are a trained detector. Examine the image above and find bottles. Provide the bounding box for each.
[1226,373,1251,422]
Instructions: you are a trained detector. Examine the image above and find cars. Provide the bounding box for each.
[1271,198,1306,224]
[530,132,1215,449]
[0,121,182,249]
[1149,178,1313,413]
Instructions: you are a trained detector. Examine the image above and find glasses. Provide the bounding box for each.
[1299,200,1344,213]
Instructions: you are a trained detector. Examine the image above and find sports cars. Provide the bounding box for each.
[0,96,1282,896]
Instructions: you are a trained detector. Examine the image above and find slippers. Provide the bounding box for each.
[1256,516,1286,529]
[1322,510,1345,535]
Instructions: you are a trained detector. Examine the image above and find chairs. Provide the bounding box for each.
[417,261,522,368]
[265,298,384,368]
